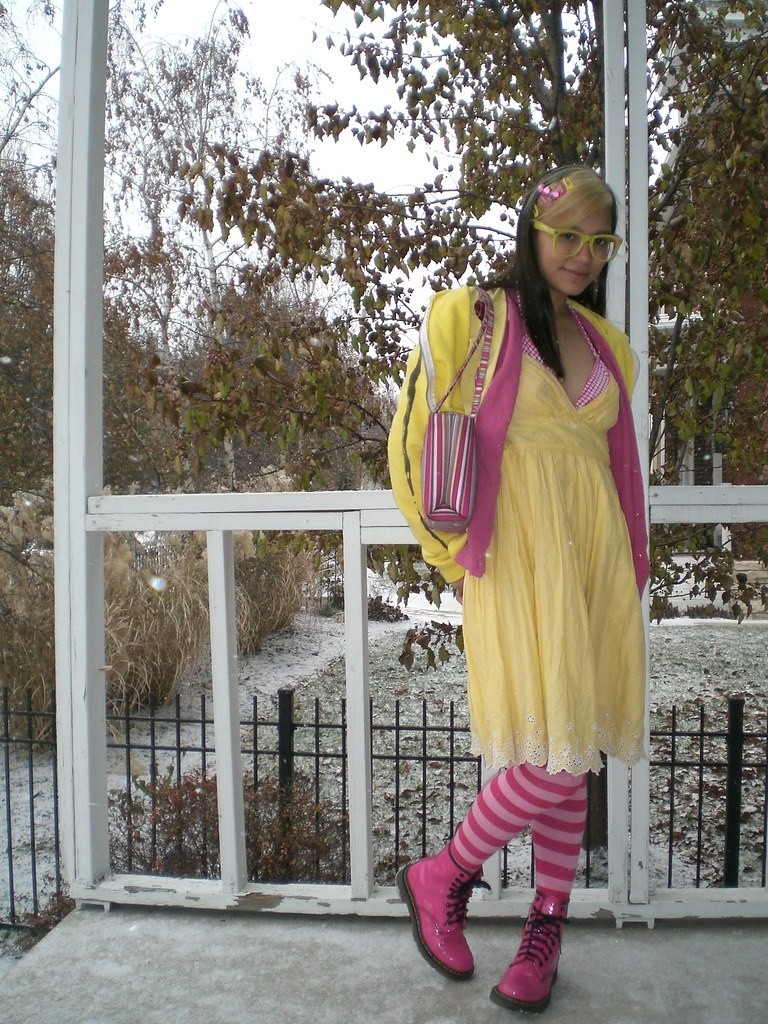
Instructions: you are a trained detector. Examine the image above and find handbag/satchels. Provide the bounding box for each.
[422,288,495,534]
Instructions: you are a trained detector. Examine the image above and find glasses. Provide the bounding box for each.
[532,219,623,263]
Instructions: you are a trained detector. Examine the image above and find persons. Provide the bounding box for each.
[393,163,650,1015]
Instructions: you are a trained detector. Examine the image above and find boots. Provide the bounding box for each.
[489,890,570,1014]
[396,842,492,981]
